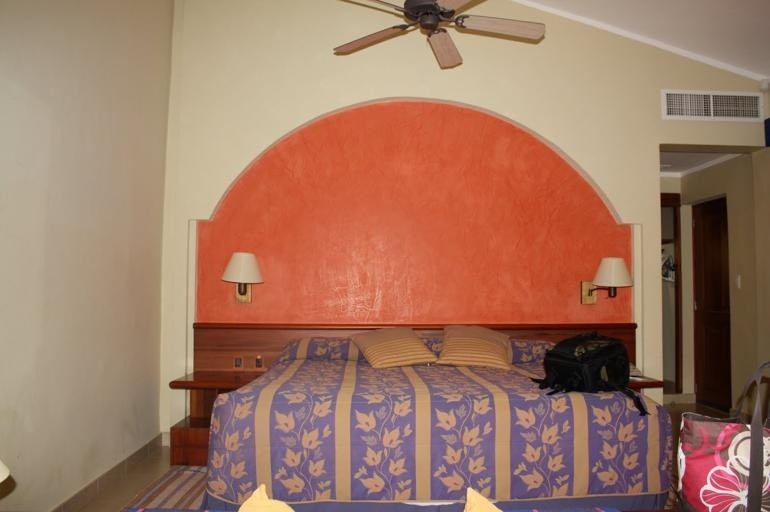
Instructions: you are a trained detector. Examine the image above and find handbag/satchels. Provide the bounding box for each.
[676,358,770,512]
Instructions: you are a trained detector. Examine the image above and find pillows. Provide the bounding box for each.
[348,326,436,369]
[437,322,515,370]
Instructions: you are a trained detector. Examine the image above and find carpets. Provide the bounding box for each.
[112,465,208,511]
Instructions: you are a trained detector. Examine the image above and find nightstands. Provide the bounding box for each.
[169,370,263,467]
[624,372,668,392]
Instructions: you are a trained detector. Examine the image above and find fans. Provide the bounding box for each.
[331,0,546,73]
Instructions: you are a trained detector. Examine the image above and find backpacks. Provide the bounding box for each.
[529,335,648,416]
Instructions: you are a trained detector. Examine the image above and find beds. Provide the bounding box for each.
[192,322,678,512]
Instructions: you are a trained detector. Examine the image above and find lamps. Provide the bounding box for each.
[221,250,263,305]
[581,256,637,306]
